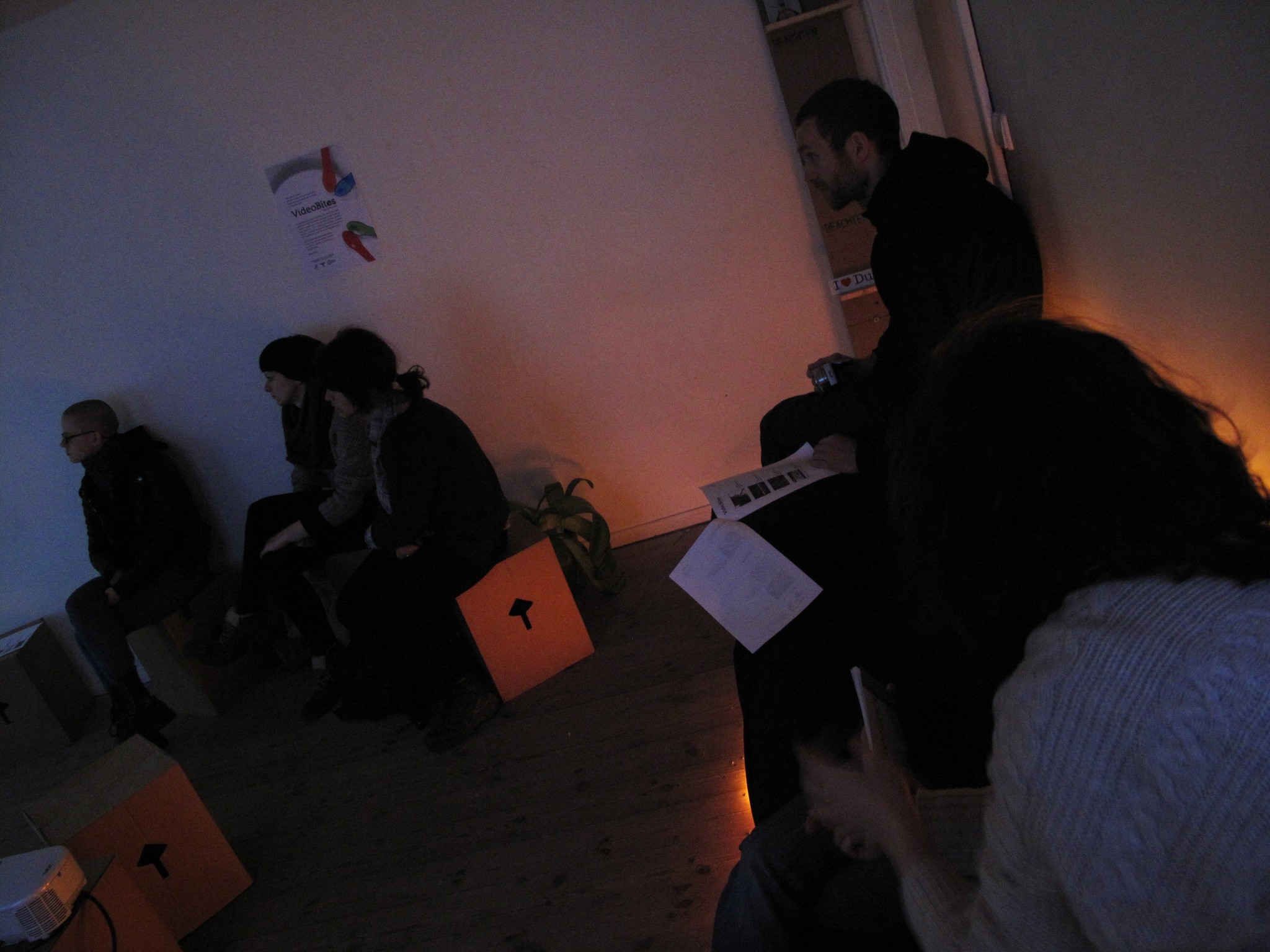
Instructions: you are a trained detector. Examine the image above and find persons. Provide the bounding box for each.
[59,397,209,754]
[200,331,378,724]
[729,75,1051,746]
[710,315,1269,952]
[304,324,516,756]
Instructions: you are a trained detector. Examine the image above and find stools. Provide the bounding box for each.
[19,733,253,944]
[0,619,91,774]
[126,621,219,717]
[454,513,595,707]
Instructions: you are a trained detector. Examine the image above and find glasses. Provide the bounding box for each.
[60,431,105,442]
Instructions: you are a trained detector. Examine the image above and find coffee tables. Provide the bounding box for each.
[3,855,181,950]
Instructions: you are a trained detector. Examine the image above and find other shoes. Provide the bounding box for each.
[198,609,257,664]
[107,704,135,746]
[300,663,353,719]
[131,693,177,748]
[429,679,489,748]
